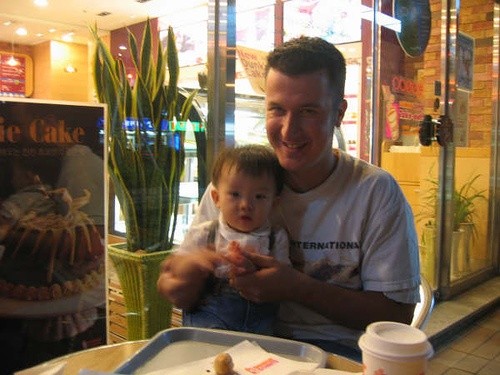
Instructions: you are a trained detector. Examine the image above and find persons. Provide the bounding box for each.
[171,145,332,337]
[157,34,420,364]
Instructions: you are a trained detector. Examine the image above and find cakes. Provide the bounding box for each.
[0,174,106,344]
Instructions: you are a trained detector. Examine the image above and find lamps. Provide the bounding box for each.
[5,41,19,66]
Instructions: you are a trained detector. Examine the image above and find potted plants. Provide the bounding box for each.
[413,162,488,286]
[91,17,201,345]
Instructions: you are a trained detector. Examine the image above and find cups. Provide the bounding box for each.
[358,321,434,375]
